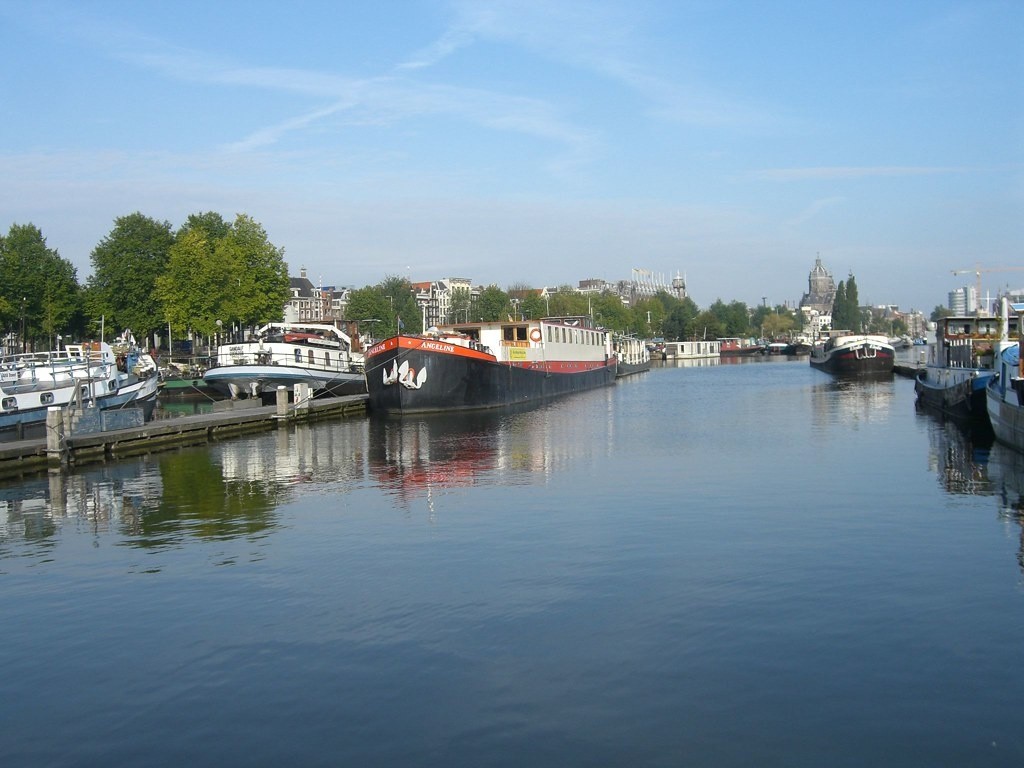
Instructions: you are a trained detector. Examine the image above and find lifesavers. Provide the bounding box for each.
[529,328,542,342]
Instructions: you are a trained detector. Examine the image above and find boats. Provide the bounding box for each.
[717,336,814,357]
[0,329,166,445]
[202,316,652,415]
[808,328,896,374]
[913,315,1024,451]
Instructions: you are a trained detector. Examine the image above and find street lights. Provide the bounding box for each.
[596,313,604,323]
[385,296,394,326]
[461,308,468,323]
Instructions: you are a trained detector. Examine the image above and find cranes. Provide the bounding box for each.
[948,257,1023,307]
